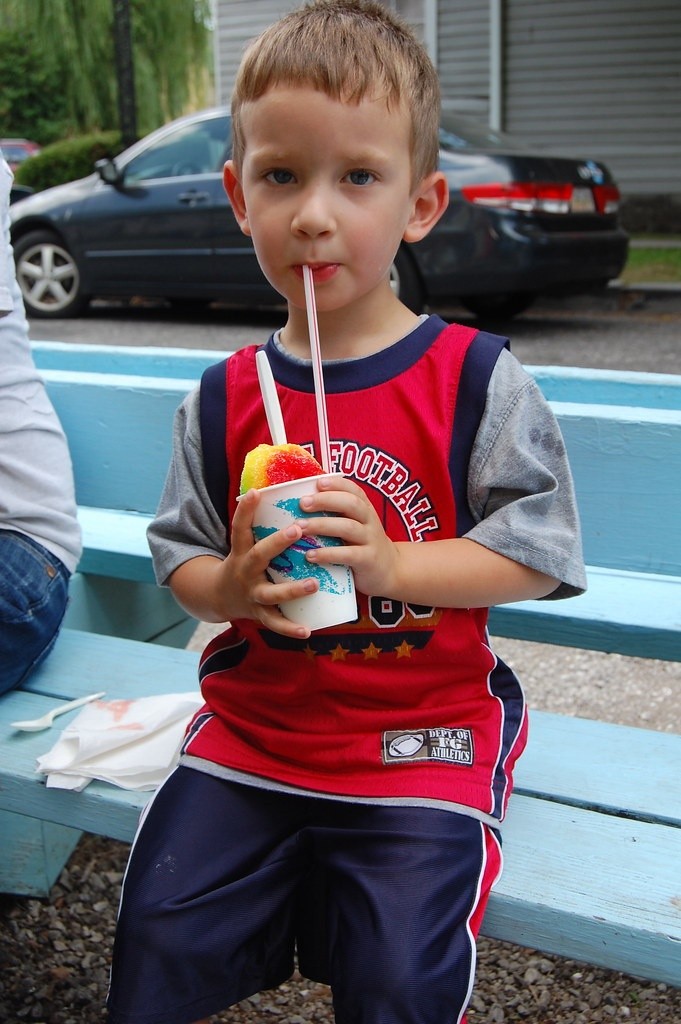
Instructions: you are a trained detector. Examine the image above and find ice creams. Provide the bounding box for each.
[237,444,328,494]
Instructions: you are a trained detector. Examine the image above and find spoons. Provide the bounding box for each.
[10,692,106,733]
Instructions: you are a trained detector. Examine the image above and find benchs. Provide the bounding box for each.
[0,340,681,986]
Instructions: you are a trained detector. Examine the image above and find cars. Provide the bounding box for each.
[0,139,43,173]
[6,107,632,326]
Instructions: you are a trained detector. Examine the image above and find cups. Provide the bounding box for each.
[237,472,358,631]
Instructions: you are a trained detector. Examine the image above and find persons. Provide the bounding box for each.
[0,152,85,698]
[103,0,587,1023]
